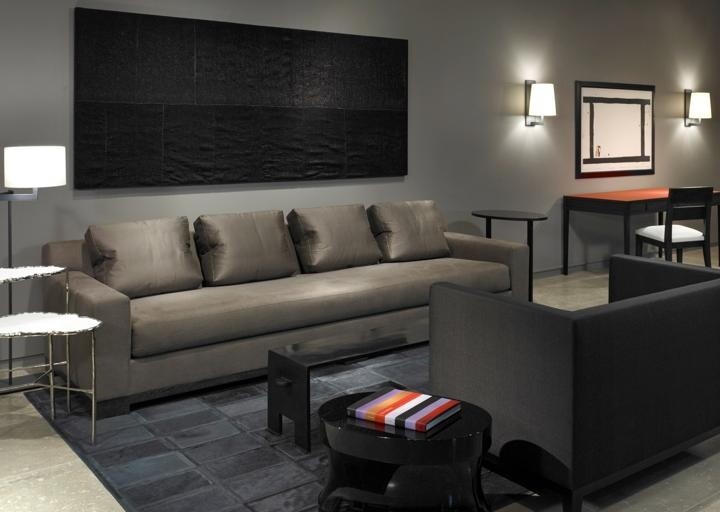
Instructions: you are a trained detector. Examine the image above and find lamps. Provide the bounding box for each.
[683,88,712,128]
[0,144,67,386]
[522,80,557,127]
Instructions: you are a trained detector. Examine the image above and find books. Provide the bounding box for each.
[346,385,463,433]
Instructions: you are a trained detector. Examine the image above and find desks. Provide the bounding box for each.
[561,186,720,275]
[316,390,582,512]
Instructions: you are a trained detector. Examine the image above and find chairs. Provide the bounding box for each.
[635,185,714,267]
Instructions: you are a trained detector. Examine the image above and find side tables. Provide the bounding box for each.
[472,210,548,300]
[0,312,103,446]
[0,263,72,413]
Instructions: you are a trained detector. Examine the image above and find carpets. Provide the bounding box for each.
[26,341,719,510]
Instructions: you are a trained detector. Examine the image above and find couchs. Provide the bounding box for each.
[42,200,529,418]
[430,254,720,495]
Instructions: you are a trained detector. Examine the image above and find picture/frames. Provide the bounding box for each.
[575,80,656,178]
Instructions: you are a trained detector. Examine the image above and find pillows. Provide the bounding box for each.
[285,203,382,273]
[192,209,298,285]
[367,200,452,260]
[83,215,204,294]
